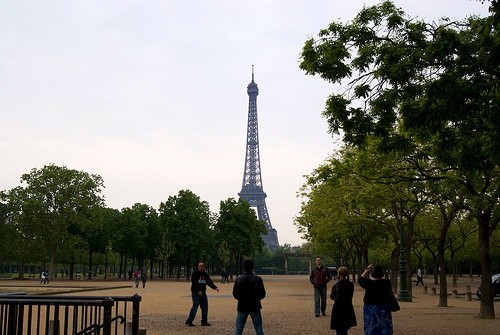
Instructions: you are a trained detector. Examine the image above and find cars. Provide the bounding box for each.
[476,274,500,299]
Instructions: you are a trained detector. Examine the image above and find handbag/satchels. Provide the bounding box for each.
[386,278,400,312]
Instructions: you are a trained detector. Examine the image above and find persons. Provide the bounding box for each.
[40,269,49,284]
[416,269,424,287]
[129,269,133,281]
[330,267,358,335]
[185,262,220,326]
[358,264,393,335]
[423,267,429,278]
[309,257,331,317]
[134,269,141,288]
[232,259,266,335]
[87,271,92,280]
[221,267,229,284]
[141,272,147,289]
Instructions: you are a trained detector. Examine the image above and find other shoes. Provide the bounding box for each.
[322,313,326,316]
[201,323,211,326]
[185,321,195,326]
[315,315,319,317]
